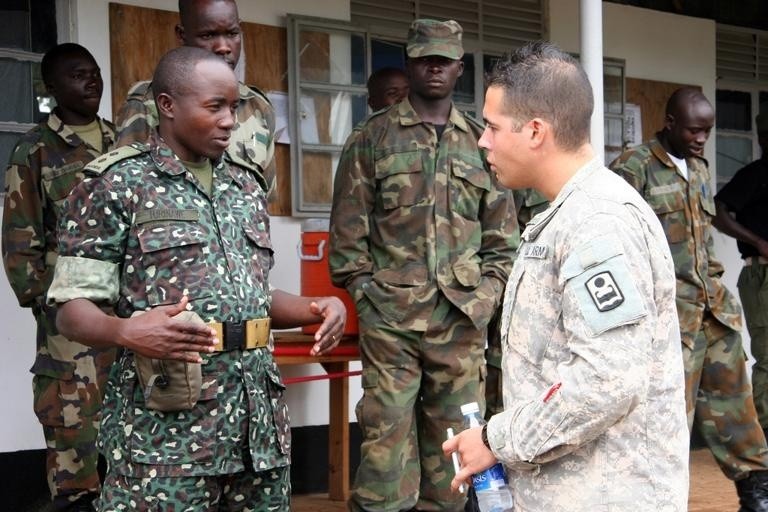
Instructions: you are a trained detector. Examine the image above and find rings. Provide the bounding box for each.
[331,336,337,342]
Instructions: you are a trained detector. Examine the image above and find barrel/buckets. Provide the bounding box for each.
[297,218,358,336]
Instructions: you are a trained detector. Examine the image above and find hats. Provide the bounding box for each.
[406,19,465,60]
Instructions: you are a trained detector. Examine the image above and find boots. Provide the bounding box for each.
[735,470,768,512]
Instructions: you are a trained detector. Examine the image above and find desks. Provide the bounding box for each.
[271,330,363,501]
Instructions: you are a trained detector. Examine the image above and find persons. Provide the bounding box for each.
[46,44,347,512]
[113,0,279,203]
[606,86,768,512]
[1,43,119,511]
[327,19,520,512]
[440,39,691,512]
[711,114,767,439]
[365,65,411,114]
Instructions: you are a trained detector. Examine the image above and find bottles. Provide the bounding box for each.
[459,401,514,512]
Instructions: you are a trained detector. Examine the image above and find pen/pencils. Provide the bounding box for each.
[446,428,465,494]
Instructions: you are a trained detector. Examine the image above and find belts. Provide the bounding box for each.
[206,317,272,352]
[747,256,768,265]
